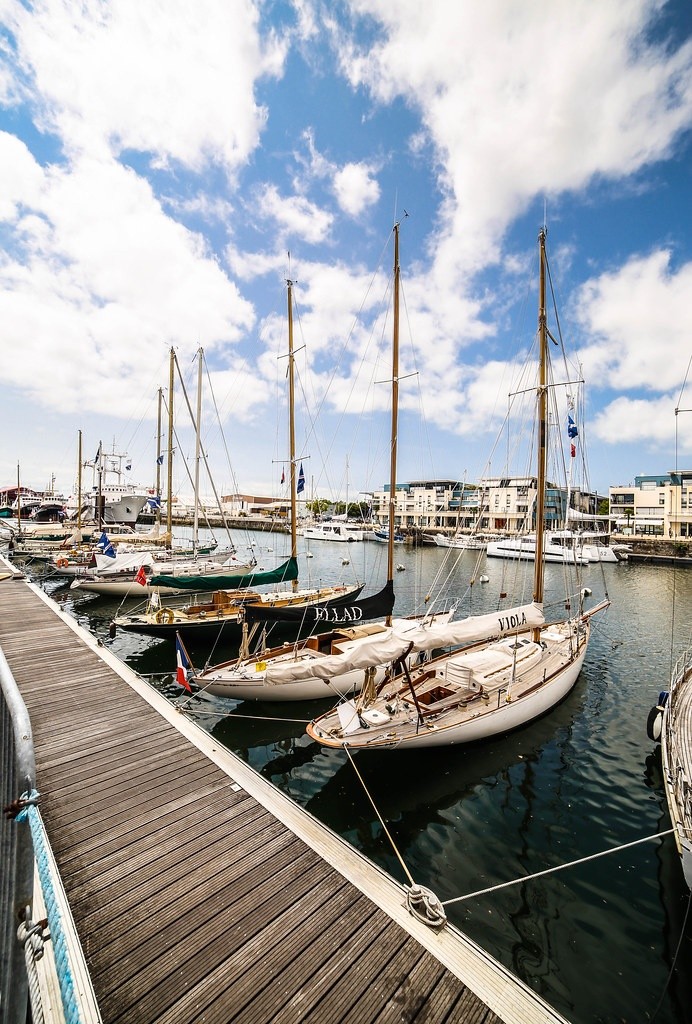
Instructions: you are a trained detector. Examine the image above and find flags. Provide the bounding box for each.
[156,455,163,465]
[136,568,146,586]
[297,464,305,494]
[175,638,191,692]
[96,532,116,558]
[571,444,575,457]
[281,468,285,483]
[126,465,131,470]
[147,497,161,508]
[568,416,578,439]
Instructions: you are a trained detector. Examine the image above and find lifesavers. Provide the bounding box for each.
[155,608,175,623]
[57,559,68,568]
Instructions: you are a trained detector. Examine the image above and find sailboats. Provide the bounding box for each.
[1,189,634,752]
